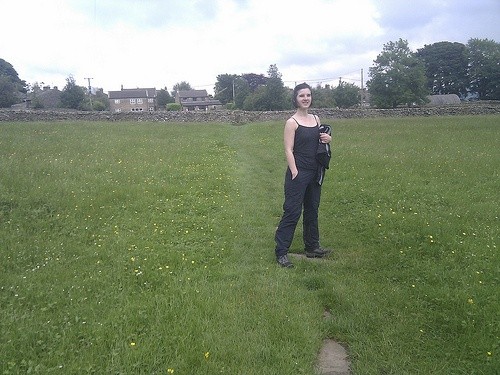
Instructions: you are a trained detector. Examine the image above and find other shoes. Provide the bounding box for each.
[276,254,294,268]
[305,246,331,258]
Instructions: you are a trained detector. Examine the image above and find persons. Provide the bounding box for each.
[274,83,332,269]
